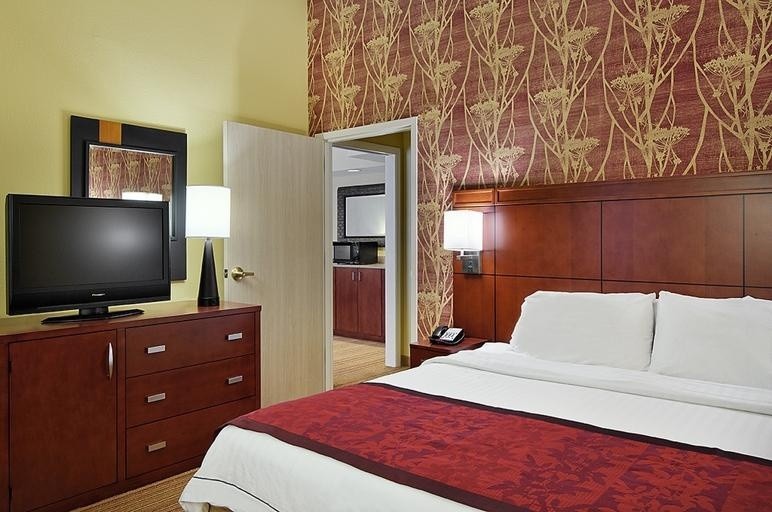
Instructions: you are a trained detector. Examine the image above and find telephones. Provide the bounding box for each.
[428,325,466,346]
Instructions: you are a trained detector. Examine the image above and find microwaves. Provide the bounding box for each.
[332,241,378,265]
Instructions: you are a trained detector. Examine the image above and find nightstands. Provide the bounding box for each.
[410,338,488,368]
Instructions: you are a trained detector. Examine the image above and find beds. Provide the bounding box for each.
[177,170,772,512]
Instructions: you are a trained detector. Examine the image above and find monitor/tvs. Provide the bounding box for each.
[5,192,170,325]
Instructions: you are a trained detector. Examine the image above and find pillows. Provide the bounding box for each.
[508,291,656,372]
[648,290,772,389]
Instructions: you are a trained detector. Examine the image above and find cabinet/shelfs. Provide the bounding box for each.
[0,322,119,512]
[333,266,385,343]
[126,305,262,493]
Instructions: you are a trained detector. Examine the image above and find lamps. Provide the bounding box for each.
[185,186,231,307]
[122,192,163,201]
[443,210,483,274]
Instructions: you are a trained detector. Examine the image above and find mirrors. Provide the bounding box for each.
[69,115,187,282]
